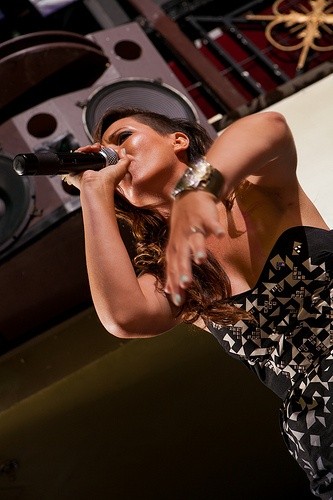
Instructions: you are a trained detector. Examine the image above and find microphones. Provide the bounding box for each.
[12,148,119,177]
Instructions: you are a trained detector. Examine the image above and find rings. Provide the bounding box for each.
[187,225,205,240]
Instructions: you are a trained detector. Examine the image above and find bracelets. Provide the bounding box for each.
[173,164,225,199]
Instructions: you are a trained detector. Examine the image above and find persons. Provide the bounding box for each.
[64,109,333,499]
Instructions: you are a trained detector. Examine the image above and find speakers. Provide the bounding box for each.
[0,21,219,262]
